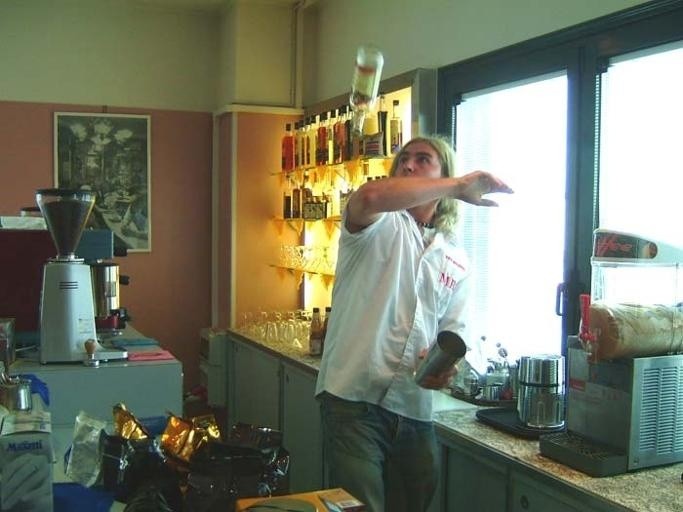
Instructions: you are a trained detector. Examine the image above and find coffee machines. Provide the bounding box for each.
[35,188,130,365]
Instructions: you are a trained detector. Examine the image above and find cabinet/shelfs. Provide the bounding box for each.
[439,443,591,512]
[268,156,395,280]
[225,338,327,494]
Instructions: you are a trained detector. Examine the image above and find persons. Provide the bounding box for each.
[309,134,515,511]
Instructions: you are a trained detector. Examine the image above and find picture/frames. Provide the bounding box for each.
[52,109,152,254]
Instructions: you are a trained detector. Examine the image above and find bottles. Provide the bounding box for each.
[280,170,333,219]
[347,45,387,135]
[322,306,334,354]
[281,96,403,171]
[309,307,323,358]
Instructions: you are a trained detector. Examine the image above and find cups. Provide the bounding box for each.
[412,330,467,392]
[242,310,312,350]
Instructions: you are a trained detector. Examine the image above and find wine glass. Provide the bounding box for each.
[279,242,335,275]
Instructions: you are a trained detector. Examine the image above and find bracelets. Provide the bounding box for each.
[410,217,438,230]
